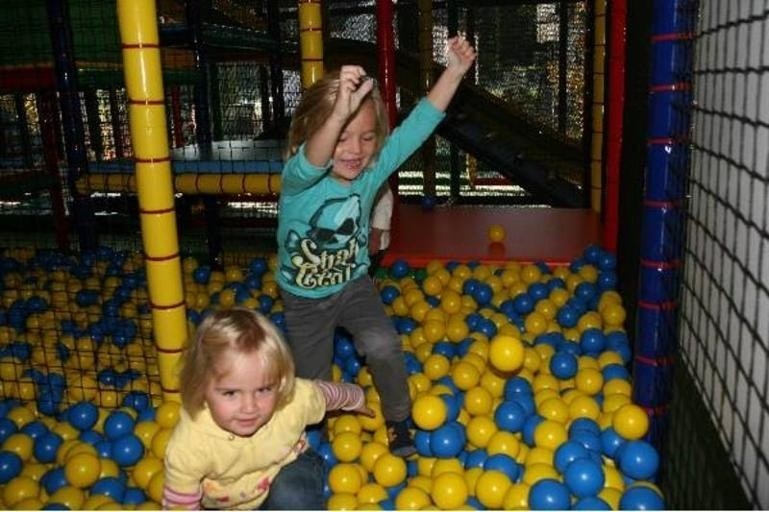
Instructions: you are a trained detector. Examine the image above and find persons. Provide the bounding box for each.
[273,33,481,462]
[359,180,395,283]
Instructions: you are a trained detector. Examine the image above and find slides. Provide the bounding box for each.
[83,0,589,212]
